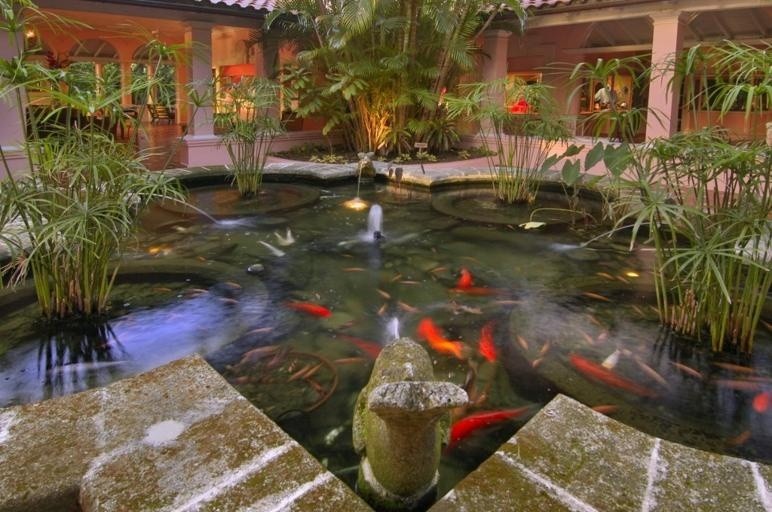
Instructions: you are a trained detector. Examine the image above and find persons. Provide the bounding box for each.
[594,86,616,110]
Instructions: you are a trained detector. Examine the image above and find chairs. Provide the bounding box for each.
[146,103,175,124]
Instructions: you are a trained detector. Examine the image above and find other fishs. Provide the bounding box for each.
[21,188,771,471]
